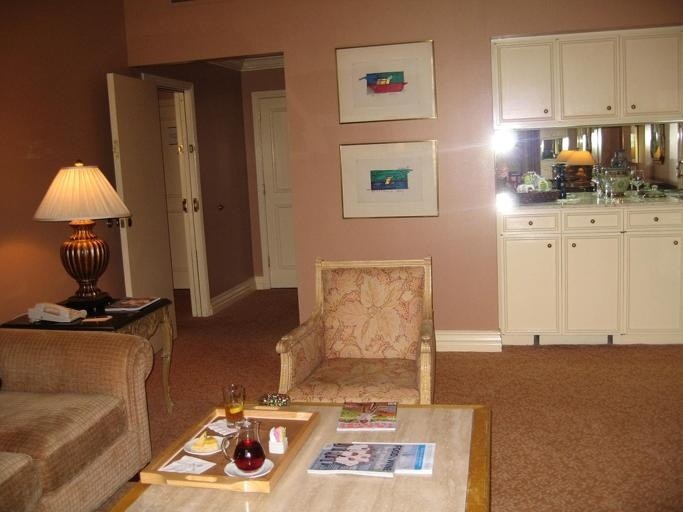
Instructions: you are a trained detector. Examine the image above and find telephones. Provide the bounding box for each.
[28,303,82,324]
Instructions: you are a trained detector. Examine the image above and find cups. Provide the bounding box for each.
[223,383,246,429]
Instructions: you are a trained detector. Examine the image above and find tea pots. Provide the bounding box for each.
[221,420,266,473]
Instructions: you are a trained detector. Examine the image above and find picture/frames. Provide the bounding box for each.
[333,38,440,221]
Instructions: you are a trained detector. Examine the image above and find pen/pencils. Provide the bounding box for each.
[95,316,109,318]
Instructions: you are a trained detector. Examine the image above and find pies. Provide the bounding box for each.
[191,431,219,454]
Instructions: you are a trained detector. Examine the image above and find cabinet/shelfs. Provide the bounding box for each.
[625,209,683,336]
[558,32,682,121]
[495,41,555,124]
[500,209,623,337]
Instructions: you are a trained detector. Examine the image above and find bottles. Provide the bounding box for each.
[555,163,567,200]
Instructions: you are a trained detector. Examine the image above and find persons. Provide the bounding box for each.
[494,155,508,189]
[358,403,376,425]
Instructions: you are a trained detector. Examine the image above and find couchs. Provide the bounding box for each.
[0,325,153,511]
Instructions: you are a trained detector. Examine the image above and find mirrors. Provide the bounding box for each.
[493,121,683,202]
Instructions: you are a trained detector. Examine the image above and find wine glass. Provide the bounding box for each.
[592,164,645,206]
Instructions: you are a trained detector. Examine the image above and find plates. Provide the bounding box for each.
[224,458,274,480]
[183,436,230,456]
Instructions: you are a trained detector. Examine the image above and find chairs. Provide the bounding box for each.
[274,254,437,405]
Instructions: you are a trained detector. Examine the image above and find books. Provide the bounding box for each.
[336,402,399,431]
[307,443,400,478]
[104,296,158,312]
[353,442,437,474]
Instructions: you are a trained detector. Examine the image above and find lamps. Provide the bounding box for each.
[566,150,596,189]
[30,158,132,314]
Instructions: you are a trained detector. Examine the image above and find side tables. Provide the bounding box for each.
[0,297,176,416]
